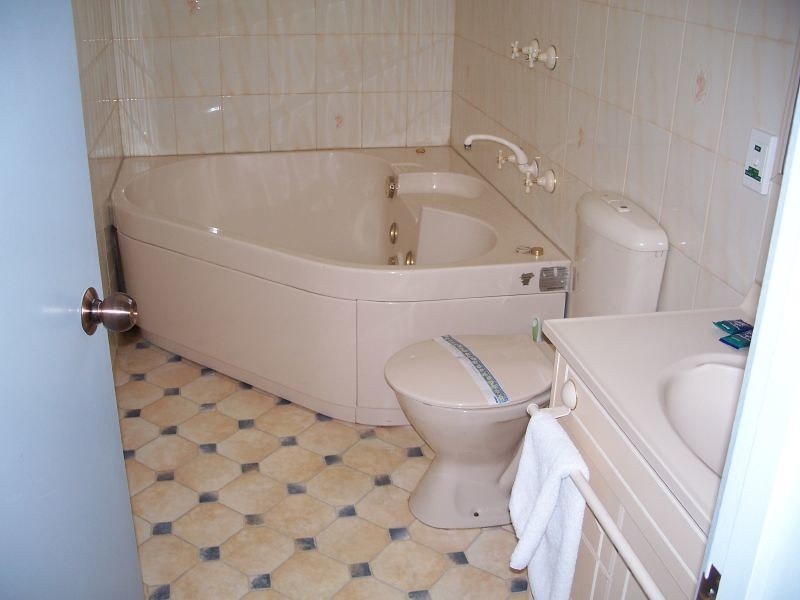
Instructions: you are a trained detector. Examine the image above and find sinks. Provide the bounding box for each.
[663,354,748,479]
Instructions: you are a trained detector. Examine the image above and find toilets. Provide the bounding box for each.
[384,189,670,529]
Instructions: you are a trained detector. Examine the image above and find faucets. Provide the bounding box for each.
[463,133,540,179]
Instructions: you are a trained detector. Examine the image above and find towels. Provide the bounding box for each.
[507,411,591,599]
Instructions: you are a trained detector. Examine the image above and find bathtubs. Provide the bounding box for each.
[110,144,573,427]
